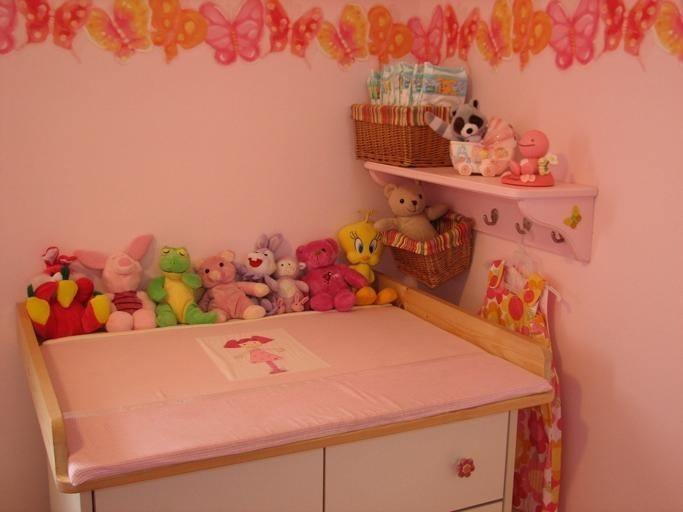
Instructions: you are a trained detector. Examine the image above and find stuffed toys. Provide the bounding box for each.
[373,179,448,243]
[146,245,219,327]
[424,97,488,143]
[235,233,282,318]
[25,246,112,340]
[338,208,399,305]
[192,248,270,323]
[296,237,368,312]
[265,256,310,314]
[73,235,157,332]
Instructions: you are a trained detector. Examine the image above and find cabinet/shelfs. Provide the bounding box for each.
[15,270,556,512]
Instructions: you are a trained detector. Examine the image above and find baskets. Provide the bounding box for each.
[383,209,475,290]
[352,103,455,167]
